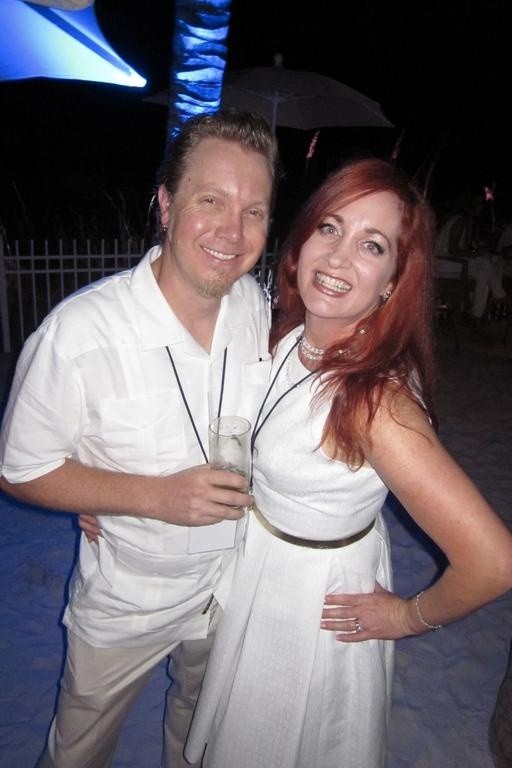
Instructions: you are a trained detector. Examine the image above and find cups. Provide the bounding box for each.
[209,416,253,511]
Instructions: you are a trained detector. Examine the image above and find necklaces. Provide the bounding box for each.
[299,334,349,362]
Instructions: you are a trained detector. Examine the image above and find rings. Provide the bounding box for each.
[354,616,367,635]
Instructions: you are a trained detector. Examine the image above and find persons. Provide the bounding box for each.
[495,216,512,283]
[70,159,512,768]
[431,209,512,341]
[0,106,276,768]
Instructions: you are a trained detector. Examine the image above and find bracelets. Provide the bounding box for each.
[411,585,447,633]
